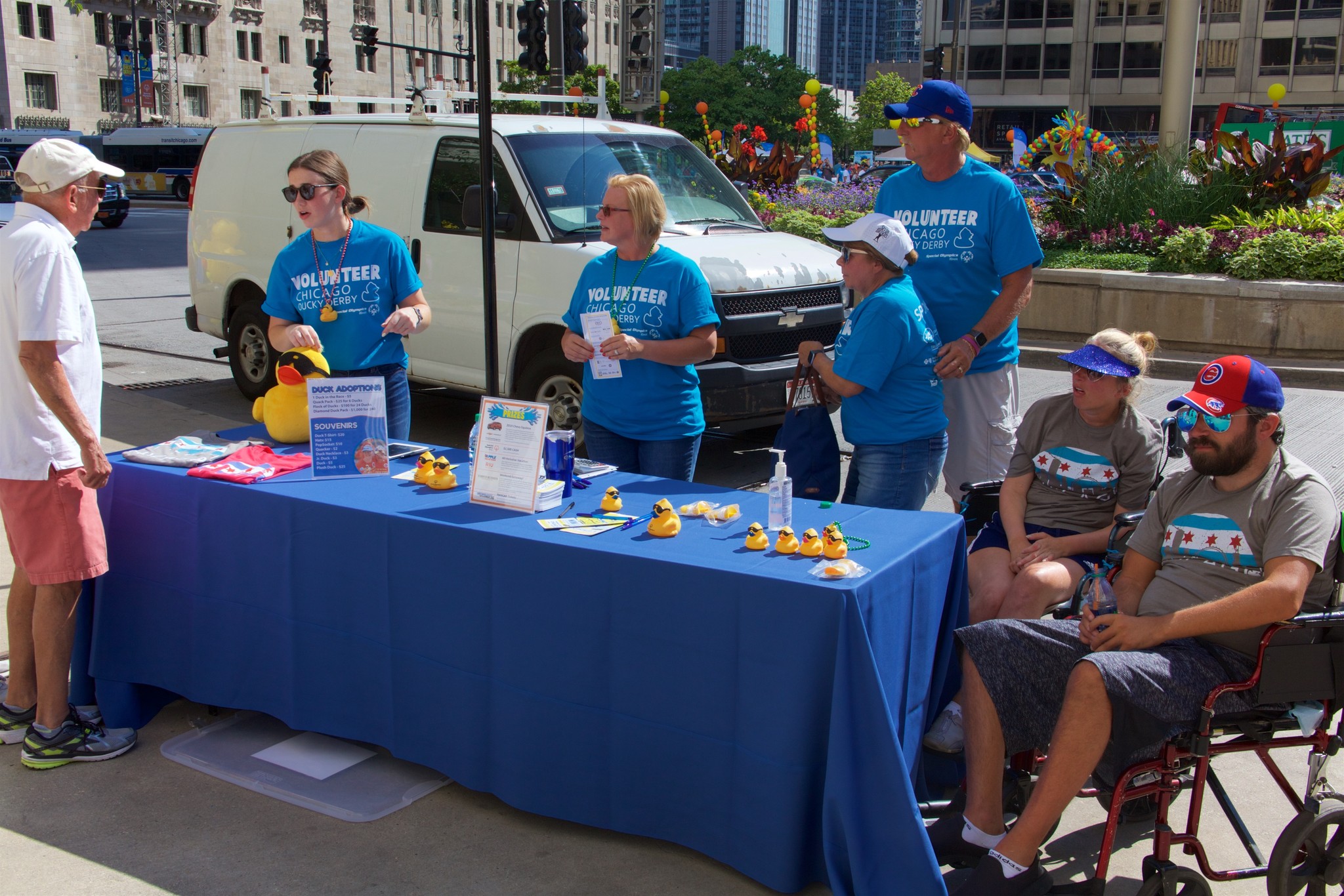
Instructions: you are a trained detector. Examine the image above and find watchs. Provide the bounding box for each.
[807,349,824,368]
[967,329,988,348]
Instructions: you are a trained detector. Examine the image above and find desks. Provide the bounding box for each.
[69,424,972,896]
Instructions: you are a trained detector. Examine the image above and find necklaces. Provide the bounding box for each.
[311,213,352,322]
[608,242,656,335]
[821,521,870,550]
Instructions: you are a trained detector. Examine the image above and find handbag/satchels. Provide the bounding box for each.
[770,360,841,503]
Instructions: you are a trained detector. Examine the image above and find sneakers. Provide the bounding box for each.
[922,699,965,752]
[20,703,137,769]
[948,850,1054,896]
[925,810,1010,855]
[0,701,102,745]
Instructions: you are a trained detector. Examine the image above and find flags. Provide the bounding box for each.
[138,53,154,108]
[120,50,136,107]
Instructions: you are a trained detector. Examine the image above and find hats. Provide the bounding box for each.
[883,78,973,132]
[1057,343,1140,378]
[13,137,125,193]
[1167,353,1286,417]
[822,212,915,271]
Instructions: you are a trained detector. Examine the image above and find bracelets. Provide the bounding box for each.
[411,306,423,326]
[959,335,980,359]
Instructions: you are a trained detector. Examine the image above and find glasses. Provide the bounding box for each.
[73,177,107,199]
[281,183,337,203]
[1066,360,1109,382]
[840,245,870,265]
[900,115,944,128]
[598,204,631,217]
[1175,406,1265,434]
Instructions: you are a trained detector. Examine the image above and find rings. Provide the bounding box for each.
[958,366,963,374]
[614,348,619,356]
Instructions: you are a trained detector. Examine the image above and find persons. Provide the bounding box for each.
[872,80,1040,518]
[807,156,897,185]
[0,137,143,767]
[923,353,1340,896]
[999,159,1053,185]
[562,173,717,481]
[919,328,1165,747]
[798,213,949,511]
[268,150,432,442]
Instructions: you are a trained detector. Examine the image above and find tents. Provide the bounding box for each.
[965,141,1001,170]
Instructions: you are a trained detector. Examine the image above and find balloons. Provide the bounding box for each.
[1006,106,1126,173]
[697,102,726,164]
[568,86,582,118]
[655,90,669,172]
[799,79,823,169]
[1267,83,1286,110]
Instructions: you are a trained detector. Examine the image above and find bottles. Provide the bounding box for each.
[1087,567,1121,651]
[469,413,481,491]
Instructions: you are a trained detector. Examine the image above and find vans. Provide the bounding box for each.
[184,87,853,454]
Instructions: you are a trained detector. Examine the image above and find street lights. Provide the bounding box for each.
[115,0,153,128]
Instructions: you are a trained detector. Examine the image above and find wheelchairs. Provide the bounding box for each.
[955,414,1190,619]
[920,514,1343,896]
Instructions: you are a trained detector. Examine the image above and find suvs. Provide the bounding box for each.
[841,165,911,191]
[1008,170,1083,206]
[0,149,130,228]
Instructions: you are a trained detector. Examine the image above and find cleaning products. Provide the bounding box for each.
[765,447,794,532]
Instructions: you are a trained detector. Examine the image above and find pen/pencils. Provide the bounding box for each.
[623,515,653,529]
[559,502,575,518]
[624,513,652,524]
[573,474,592,484]
[577,513,632,520]
[572,480,584,489]
[400,448,437,459]
[572,480,587,488]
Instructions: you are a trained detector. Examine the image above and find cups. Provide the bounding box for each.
[543,430,576,498]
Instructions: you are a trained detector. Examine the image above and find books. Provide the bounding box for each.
[534,456,645,536]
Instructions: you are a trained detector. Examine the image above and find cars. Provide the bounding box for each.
[796,174,836,193]
[0,180,24,229]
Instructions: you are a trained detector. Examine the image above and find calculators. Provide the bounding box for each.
[574,459,609,474]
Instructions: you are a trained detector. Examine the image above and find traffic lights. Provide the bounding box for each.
[922,43,945,80]
[563,0,589,76]
[516,0,547,71]
[312,58,334,95]
[362,26,379,56]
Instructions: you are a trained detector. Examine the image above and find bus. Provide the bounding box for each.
[103,127,213,202]
[0,128,104,161]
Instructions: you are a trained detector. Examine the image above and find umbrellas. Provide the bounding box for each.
[874,146,913,161]
